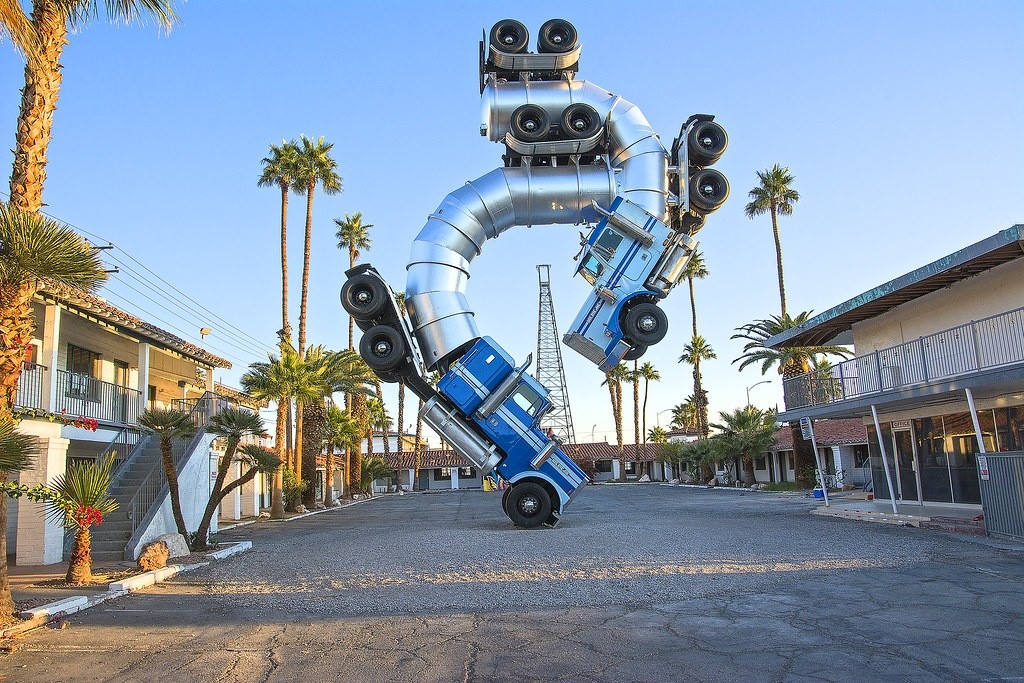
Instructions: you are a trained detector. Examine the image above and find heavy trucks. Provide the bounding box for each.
[563,113,732,374]
[340,263,591,528]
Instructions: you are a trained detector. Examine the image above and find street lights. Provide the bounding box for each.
[747,380,771,405]
[656,408,676,427]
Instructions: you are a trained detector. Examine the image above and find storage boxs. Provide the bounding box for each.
[813,486,828,498]
